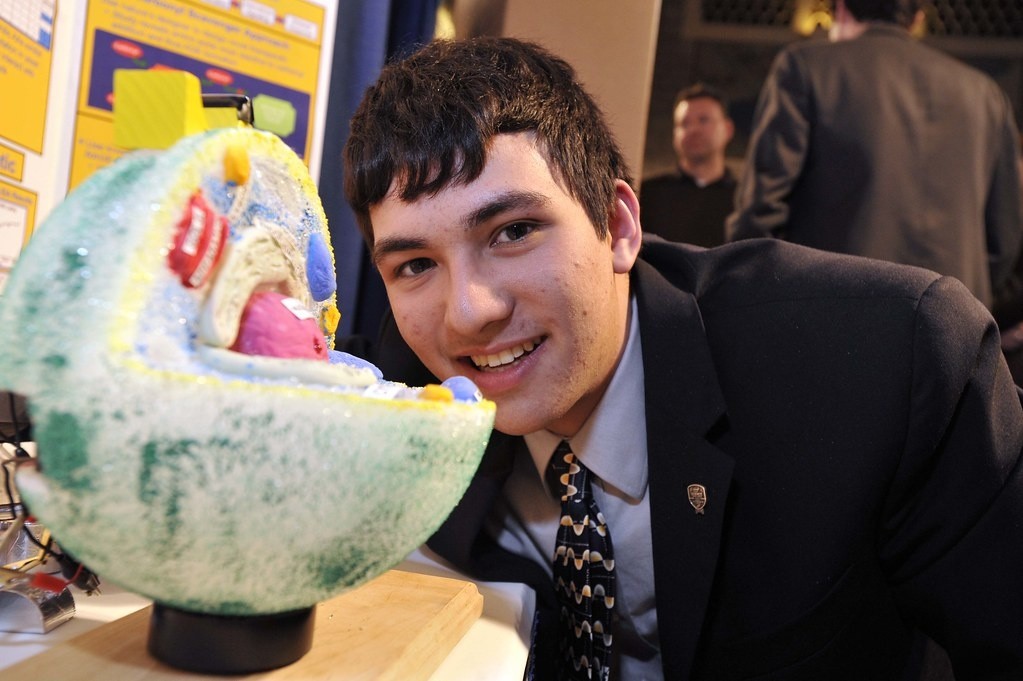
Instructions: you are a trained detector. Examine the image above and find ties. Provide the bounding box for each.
[549,439,620,681]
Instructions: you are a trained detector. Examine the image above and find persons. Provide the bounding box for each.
[725,0,1023,388]
[345,34,1023,680]
[639,83,741,248]
